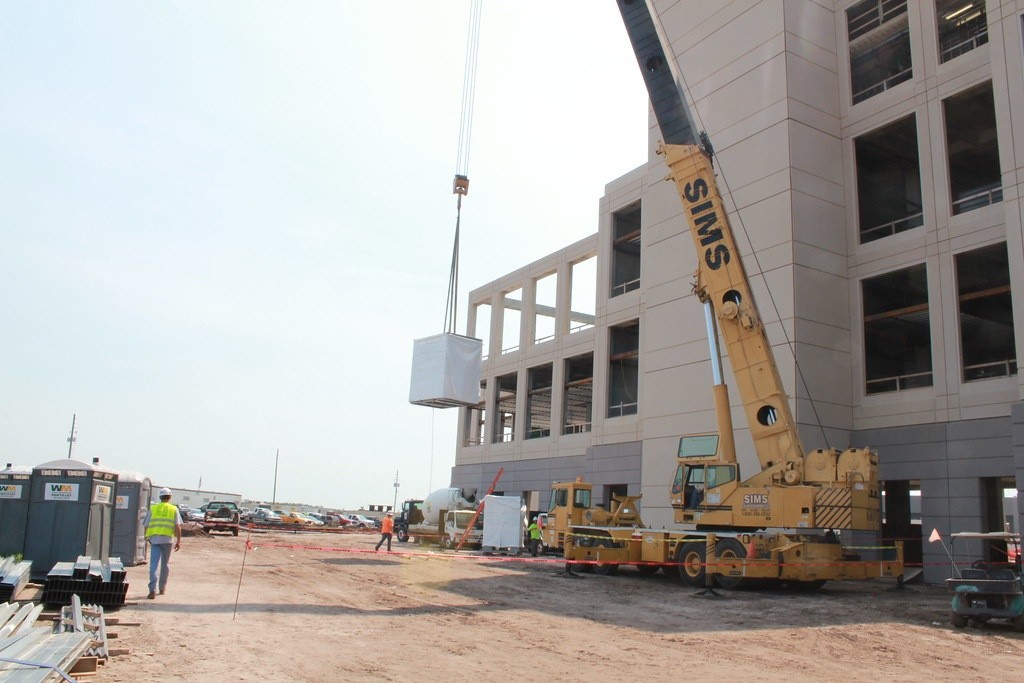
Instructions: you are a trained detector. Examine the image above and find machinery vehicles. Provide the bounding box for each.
[409,0,908,595]
[946,532,1024,631]
[537,478,647,555]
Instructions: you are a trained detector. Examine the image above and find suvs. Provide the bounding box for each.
[203,501,243,538]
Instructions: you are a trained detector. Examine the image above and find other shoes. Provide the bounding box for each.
[159,589,165,595]
[148,591,156,599]
[375,546,379,550]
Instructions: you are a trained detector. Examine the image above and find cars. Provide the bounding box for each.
[175,504,383,530]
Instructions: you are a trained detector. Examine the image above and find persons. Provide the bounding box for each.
[528,517,542,556]
[375,510,393,552]
[144,487,183,599]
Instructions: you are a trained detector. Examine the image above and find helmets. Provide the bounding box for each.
[386,510,394,516]
[159,488,173,497]
[533,517,538,521]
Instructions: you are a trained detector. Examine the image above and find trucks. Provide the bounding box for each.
[393,508,483,548]
